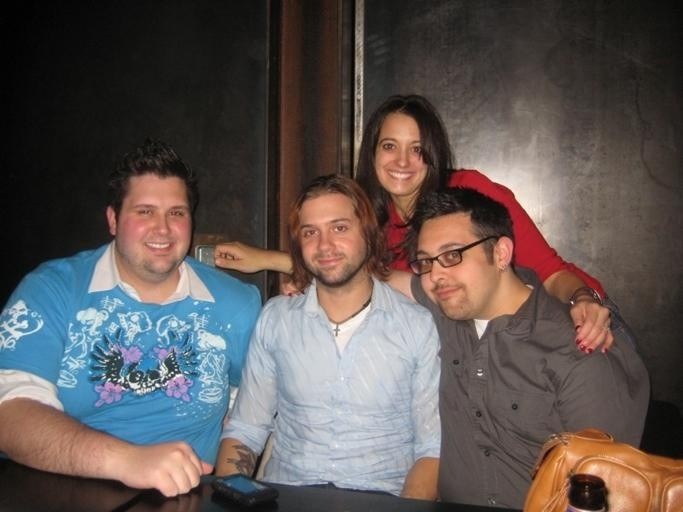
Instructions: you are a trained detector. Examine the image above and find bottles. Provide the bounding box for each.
[566,473,609,512]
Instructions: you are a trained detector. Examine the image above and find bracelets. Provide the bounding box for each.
[569,286,603,308]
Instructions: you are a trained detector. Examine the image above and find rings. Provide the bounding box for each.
[601,326,611,335]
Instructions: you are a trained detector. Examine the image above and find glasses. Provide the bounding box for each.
[409,235,498,275]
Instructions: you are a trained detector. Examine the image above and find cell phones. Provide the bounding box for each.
[212,472,278,504]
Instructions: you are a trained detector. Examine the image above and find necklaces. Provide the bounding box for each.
[330,295,372,336]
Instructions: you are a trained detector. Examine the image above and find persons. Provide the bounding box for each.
[279,94,614,354]
[0,136,263,498]
[217,174,442,502]
[214,185,651,511]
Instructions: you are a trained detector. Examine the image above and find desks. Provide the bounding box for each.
[1,451,524,512]
